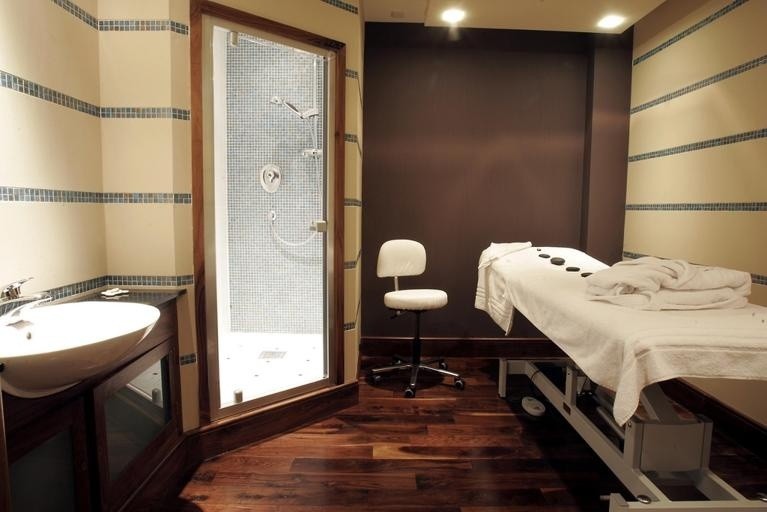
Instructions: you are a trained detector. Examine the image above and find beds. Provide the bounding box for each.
[475,242,767,512]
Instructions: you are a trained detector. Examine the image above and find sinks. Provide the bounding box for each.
[0,301,162,399]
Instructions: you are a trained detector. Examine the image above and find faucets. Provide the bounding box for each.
[0,272,51,323]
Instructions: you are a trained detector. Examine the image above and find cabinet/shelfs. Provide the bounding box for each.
[0,299,185,512]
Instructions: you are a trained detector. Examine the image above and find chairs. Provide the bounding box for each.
[365,240,465,398]
